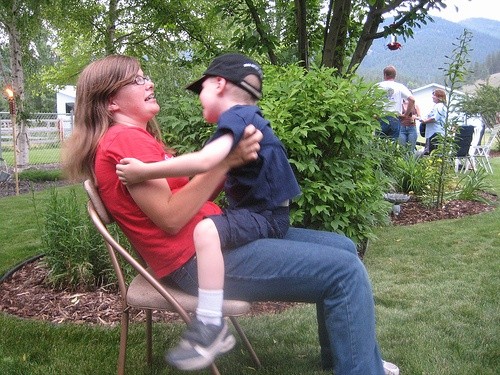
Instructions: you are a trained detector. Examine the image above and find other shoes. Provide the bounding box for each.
[380,358,400,375]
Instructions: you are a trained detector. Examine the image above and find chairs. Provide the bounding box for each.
[83,177,262,375]
[451,124,498,174]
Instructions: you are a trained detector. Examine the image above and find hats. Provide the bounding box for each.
[185,54,263,100]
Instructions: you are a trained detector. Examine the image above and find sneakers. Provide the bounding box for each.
[165,318,236,370]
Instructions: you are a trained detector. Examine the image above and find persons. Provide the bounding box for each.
[60,55,400,375]
[419,89,448,155]
[401,90,421,152]
[372,66,415,144]
[115,52,301,370]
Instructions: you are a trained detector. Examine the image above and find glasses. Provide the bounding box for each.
[122,75,152,88]
